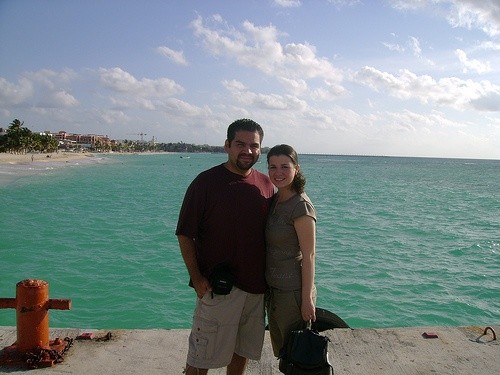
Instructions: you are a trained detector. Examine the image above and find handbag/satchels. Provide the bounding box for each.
[210,263,235,299]
[277,318,334,375]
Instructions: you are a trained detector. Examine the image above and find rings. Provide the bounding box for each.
[309,314,313,319]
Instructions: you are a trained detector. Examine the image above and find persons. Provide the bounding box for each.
[30,154,34,161]
[268,145,317,358]
[176,120,275,374]
